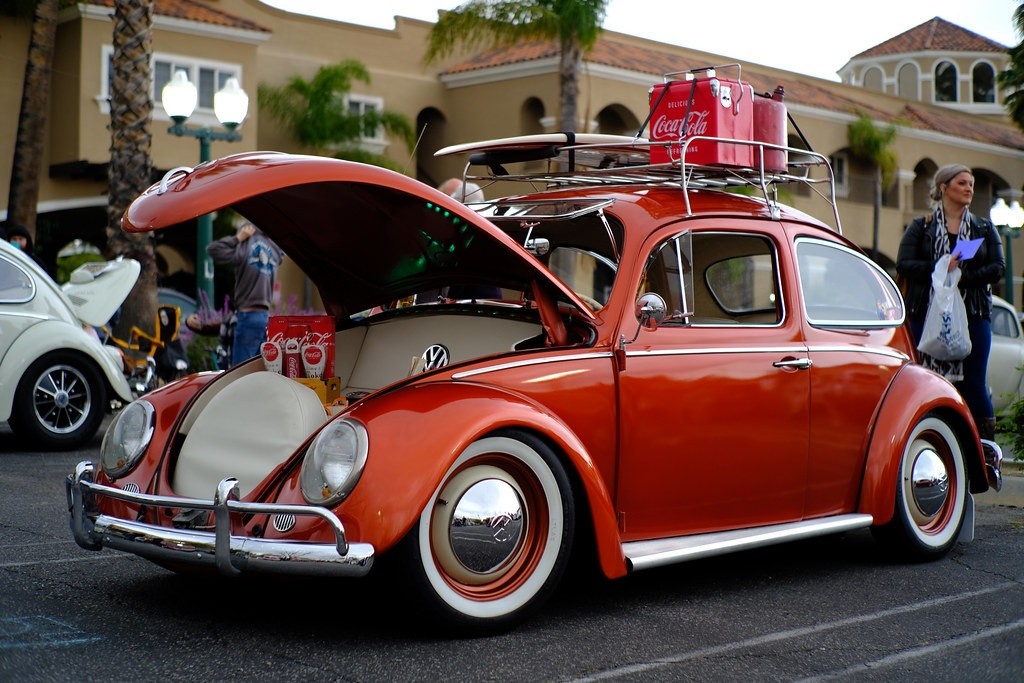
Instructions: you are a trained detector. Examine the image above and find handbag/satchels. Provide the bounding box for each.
[916,254,972,362]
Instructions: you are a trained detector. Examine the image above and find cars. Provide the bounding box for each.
[0,241,233,455]
[984,292,1024,424]
[64,132,1005,640]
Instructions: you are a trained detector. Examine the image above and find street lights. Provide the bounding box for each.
[159,66,250,308]
[989,195,1024,307]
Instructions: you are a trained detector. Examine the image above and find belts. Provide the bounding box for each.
[238,306,269,312]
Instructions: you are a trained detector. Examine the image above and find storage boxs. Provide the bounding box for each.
[647,78,753,167]
[293,375,343,404]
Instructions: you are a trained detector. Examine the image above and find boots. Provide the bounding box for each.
[978,416,996,440]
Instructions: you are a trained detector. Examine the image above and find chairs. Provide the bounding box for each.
[103,304,182,379]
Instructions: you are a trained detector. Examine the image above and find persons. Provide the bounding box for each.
[5,226,55,281]
[896,164,1005,443]
[415,177,501,306]
[206,222,287,368]
